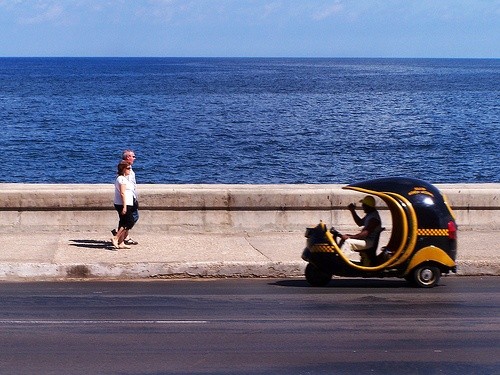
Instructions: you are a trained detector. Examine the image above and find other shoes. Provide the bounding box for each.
[110,237,119,248]
[111,229,118,236]
[114,241,131,249]
[124,238,138,245]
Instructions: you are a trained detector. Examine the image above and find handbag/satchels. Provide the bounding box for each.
[128,196,138,212]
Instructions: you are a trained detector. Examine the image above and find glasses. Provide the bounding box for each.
[131,156,135,158]
[126,167,130,169]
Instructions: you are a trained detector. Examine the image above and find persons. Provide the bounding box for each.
[337,196,381,263]
[109,150,141,249]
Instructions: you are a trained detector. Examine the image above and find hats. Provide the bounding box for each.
[358,196,378,208]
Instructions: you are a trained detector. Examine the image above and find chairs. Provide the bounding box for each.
[354,227,385,267]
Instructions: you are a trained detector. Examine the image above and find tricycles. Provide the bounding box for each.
[300,177,459,289]
